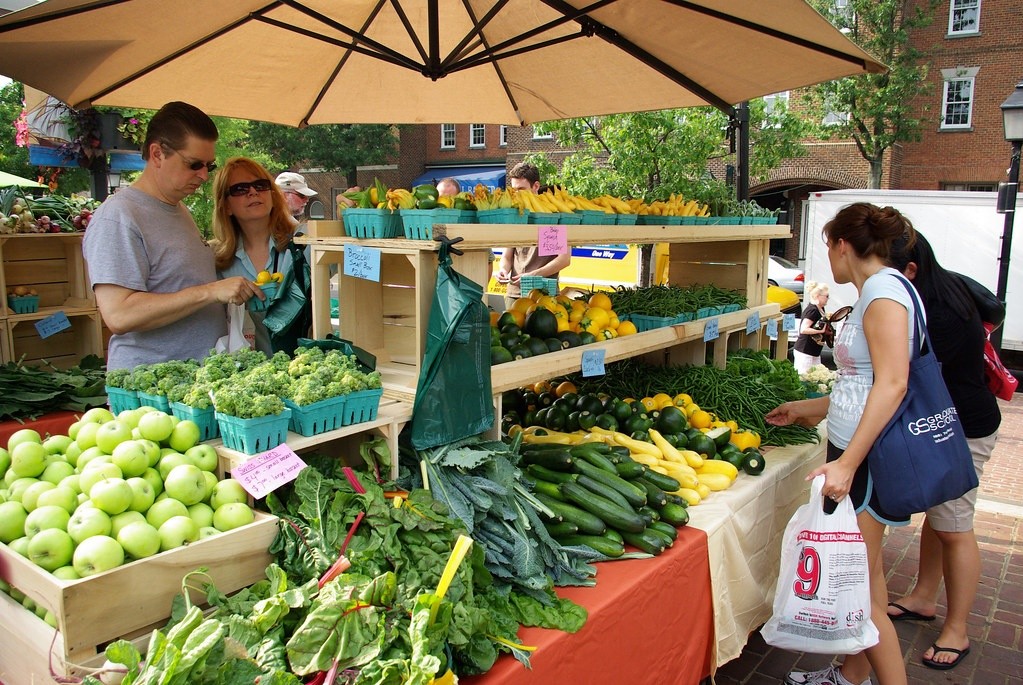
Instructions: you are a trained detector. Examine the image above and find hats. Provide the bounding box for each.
[275,172,318,196]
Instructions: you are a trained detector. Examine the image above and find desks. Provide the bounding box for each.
[684,418,831,685]
[463,526,714,685]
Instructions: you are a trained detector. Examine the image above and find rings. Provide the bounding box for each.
[832,494,837,500]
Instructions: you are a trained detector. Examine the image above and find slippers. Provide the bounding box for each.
[922,643,971,670]
[888,602,936,621]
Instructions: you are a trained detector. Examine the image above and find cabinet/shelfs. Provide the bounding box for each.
[298,212,794,444]
[0,388,506,685]
[637,311,793,369]
[0,226,104,368]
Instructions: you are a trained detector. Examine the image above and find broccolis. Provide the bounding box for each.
[798,363,840,395]
[105,345,383,417]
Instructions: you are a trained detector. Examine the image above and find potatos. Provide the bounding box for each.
[7,286,40,298]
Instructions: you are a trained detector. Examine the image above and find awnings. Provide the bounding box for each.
[411,167,506,195]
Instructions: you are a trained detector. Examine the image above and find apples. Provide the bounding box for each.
[0,403,252,630]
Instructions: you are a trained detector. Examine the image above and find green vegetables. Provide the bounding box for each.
[0,351,109,424]
[104,436,606,685]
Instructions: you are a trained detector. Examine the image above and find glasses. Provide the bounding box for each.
[291,192,305,199]
[225,178,272,198]
[172,149,217,172]
[822,295,828,298]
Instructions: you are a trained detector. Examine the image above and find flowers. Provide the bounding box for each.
[13,98,139,194]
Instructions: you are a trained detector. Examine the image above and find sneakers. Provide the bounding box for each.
[783,654,872,685]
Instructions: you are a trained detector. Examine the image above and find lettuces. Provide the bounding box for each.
[705,348,809,402]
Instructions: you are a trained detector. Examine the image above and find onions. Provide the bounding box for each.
[0,198,94,235]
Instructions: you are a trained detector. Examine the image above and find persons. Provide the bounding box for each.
[435,178,495,284]
[793,281,833,376]
[765,202,927,685]
[205,157,313,360]
[81,101,266,412]
[496,164,570,311]
[275,172,318,218]
[885,227,1004,669]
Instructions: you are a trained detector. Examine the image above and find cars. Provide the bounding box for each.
[768,255,804,294]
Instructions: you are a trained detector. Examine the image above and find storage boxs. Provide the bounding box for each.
[559,213,582,225]
[660,216,682,225]
[680,216,696,225]
[635,215,648,225]
[344,387,383,426]
[616,214,638,225]
[695,217,708,225]
[601,214,618,225]
[8,293,40,314]
[708,217,778,225]
[216,406,293,457]
[282,394,346,438]
[346,207,398,238]
[106,385,140,416]
[647,216,661,225]
[575,210,605,225]
[136,389,173,416]
[475,209,491,224]
[400,208,461,240]
[527,211,560,225]
[490,208,529,224]
[458,210,477,224]
[169,399,221,443]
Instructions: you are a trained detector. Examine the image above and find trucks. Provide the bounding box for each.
[804,189,1023,394]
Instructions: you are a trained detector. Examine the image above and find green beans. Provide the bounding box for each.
[571,283,751,321]
[553,356,822,451]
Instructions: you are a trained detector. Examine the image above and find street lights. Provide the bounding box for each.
[990,82,1023,359]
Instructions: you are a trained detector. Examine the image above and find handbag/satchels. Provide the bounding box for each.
[867,273,978,517]
[821,342,838,371]
[760,474,880,654]
[983,321,1019,402]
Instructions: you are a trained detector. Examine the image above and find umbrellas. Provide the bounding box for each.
[0,0,891,134]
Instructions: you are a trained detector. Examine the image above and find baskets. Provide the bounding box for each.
[8,296,40,314]
[104,385,381,455]
[342,207,778,239]
